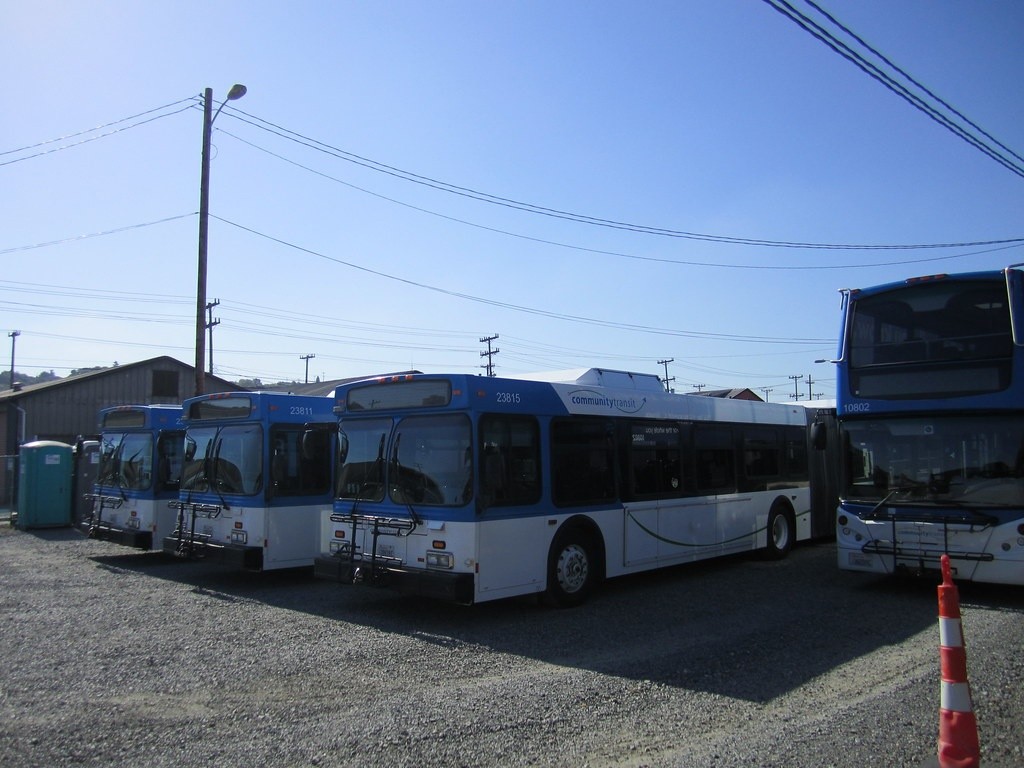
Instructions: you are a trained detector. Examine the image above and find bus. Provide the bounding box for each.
[829,262,1023,593]
[83,404,185,551]
[163,390,340,573]
[328,366,837,613]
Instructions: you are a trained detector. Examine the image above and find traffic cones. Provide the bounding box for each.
[936,554,980,768]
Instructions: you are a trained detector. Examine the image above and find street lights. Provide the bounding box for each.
[195,84,248,396]
